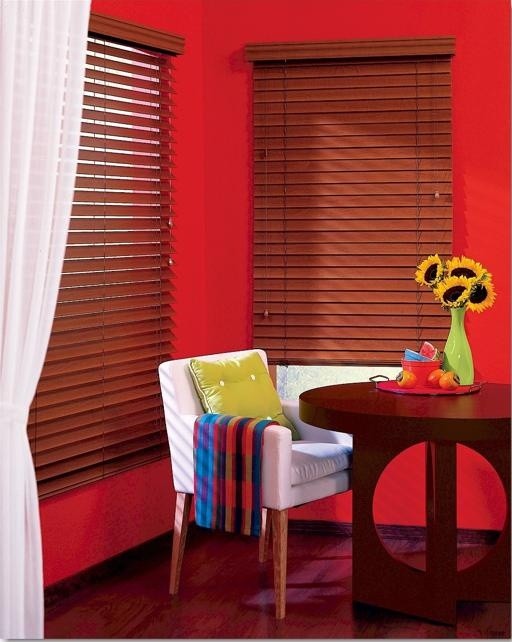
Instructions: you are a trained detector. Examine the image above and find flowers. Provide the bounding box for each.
[414,253,498,313]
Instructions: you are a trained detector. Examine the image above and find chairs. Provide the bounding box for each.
[157,347,354,621]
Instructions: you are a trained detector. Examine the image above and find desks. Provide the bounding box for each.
[298,378,511,626]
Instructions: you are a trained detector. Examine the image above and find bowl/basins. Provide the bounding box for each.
[401,360,443,386]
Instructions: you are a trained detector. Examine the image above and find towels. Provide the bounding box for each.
[193,413,280,538]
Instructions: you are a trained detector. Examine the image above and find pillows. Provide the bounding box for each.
[188,351,302,441]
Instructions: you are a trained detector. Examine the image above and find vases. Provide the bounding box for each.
[441,304,475,386]
